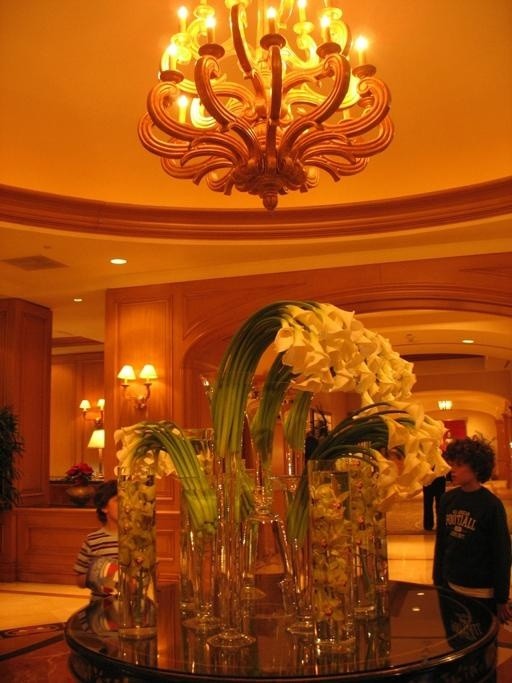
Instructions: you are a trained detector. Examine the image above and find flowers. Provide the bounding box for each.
[110,288,454,650]
[62,461,92,482]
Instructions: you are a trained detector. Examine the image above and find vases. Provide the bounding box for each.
[67,483,99,505]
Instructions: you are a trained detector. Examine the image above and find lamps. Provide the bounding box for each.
[87,428,107,481]
[134,0,394,222]
[114,356,162,424]
[78,395,106,427]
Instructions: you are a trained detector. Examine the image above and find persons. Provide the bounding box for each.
[432,436,512,626]
[72,478,120,604]
[422,472,447,529]
[444,431,455,450]
[304,419,327,462]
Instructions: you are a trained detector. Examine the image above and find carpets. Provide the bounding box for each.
[1,601,512,682]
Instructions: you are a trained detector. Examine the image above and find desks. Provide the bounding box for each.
[62,567,498,682]
